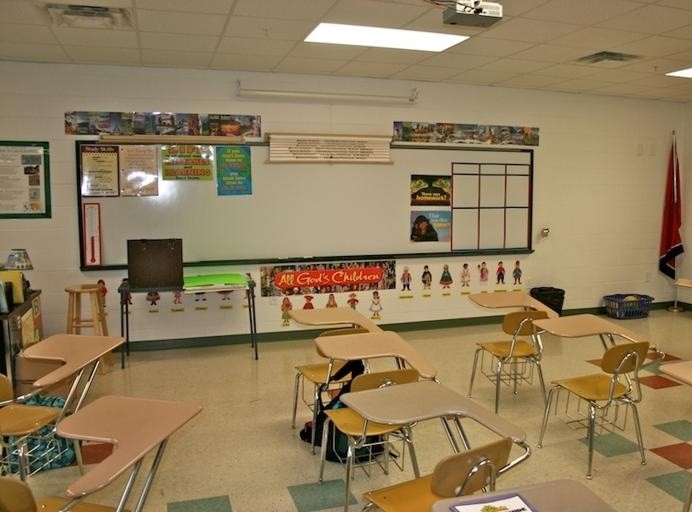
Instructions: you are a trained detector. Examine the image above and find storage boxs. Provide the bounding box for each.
[14,339,77,405]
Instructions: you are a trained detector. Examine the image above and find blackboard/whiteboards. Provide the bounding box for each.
[74,138,533,271]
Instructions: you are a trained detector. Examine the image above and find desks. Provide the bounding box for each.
[287,306,383,334]
[338,378,527,446]
[116,272,259,370]
[467,291,560,321]
[54,393,204,499]
[530,313,657,352]
[427,477,618,511]
[314,329,439,381]
[656,357,692,392]
[22,333,128,389]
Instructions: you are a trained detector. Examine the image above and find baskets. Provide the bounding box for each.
[604,293,654,320]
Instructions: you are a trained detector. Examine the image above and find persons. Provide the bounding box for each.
[280,291,383,324]
[97,273,256,307]
[400,260,522,290]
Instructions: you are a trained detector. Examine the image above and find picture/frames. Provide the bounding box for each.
[0,140,51,219]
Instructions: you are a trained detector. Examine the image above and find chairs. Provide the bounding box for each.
[361,434,513,511]
[291,328,370,456]
[466,310,548,415]
[1,373,86,479]
[0,474,131,512]
[536,340,651,481]
[317,366,422,511]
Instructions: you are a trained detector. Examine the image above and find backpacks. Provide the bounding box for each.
[300,359,382,462]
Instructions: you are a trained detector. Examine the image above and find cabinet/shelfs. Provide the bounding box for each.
[0,289,43,388]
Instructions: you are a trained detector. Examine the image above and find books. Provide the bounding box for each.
[449,493,539,512]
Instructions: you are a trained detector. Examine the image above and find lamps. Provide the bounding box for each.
[236,87,417,105]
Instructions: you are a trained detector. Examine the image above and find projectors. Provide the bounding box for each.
[443,0,502,28]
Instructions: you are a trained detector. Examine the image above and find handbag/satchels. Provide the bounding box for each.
[7,394,74,473]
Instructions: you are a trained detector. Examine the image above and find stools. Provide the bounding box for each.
[63,282,114,377]
